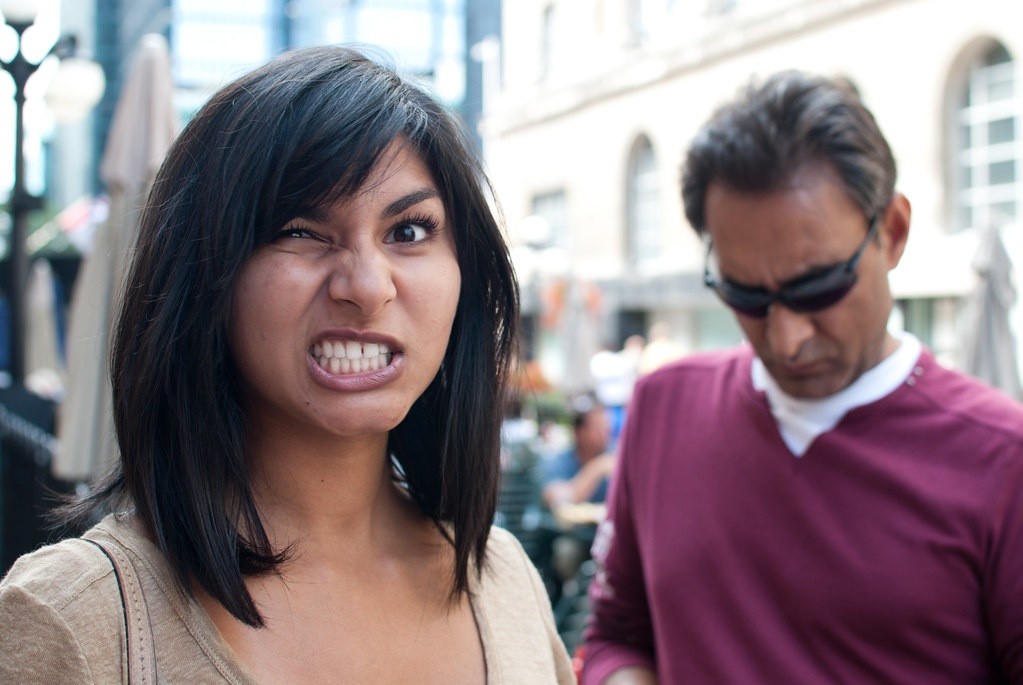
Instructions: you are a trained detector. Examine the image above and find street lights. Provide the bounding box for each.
[0,0,106,410]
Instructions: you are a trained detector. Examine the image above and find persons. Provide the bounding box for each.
[492,394,615,596]
[579,69,1023,685]
[0,47,579,685]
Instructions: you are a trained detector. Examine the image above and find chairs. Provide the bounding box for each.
[493,446,565,603]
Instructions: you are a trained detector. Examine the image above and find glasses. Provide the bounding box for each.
[701,203,885,320]
